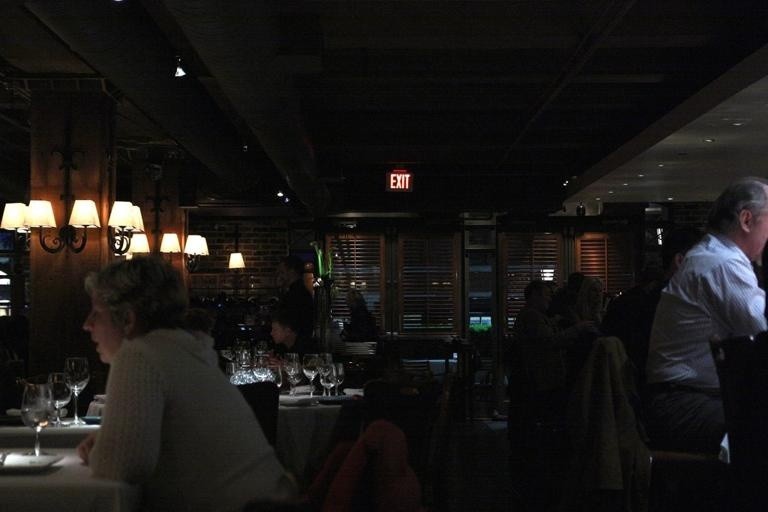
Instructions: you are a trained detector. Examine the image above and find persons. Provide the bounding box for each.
[206,307,231,345]
[184,308,223,357]
[642,235,696,295]
[634,176,768,453]
[339,290,372,342]
[271,255,314,338]
[262,320,308,376]
[76,254,300,512]
[513,272,609,339]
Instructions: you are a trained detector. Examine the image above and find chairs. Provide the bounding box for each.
[709,331,766,510]
[238,381,279,456]
[567,339,720,512]
[363,373,432,512]
[297,416,421,511]
[413,369,461,505]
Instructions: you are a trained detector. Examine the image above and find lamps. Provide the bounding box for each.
[114,233,151,261]
[115,206,145,254]
[227,251,246,271]
[159,232,182,264]
[185,234,210,273]
[107,200,132,251]
[0,202,32,255]
[27,200,65,254]
[65,199,102,254]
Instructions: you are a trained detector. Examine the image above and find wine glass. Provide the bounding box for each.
[48,372,71,426]
[317,352,345,397]
[22,382,55,460]
[302,354,320,396]
[63,354,90,426]
[231,339,271,382]
[283,353,302,395]
